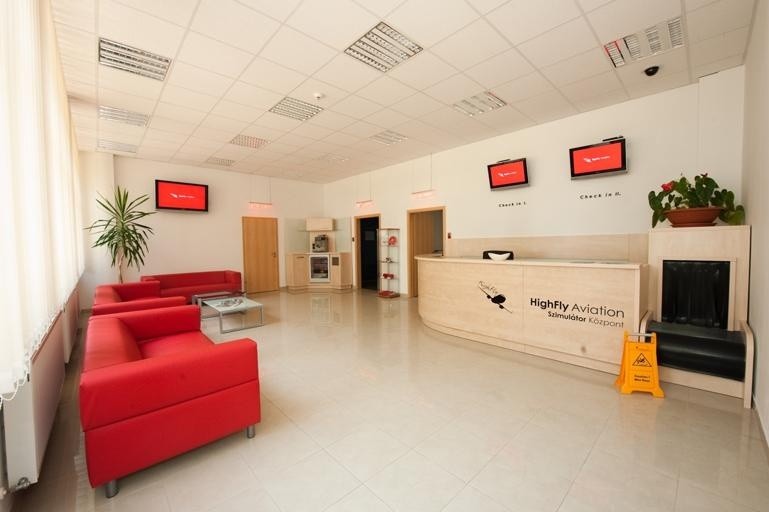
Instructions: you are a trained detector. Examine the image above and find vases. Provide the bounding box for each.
[662,207,722,226]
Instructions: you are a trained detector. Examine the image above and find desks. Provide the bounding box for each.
[200,296,265,333]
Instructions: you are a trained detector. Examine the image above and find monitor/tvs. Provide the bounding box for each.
[155,179,209,212]
[568,137,629,180]
[487,157,530,190]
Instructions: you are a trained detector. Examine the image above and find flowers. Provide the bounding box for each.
[650,173,746,226]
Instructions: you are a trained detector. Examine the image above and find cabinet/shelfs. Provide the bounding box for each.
[330,252,352,289]
[286,253,310,289]
[376,228,400,298]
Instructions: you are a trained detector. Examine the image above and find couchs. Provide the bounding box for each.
[91,281,187,315]
[141,270,242,300]
[79,304,261,499]
